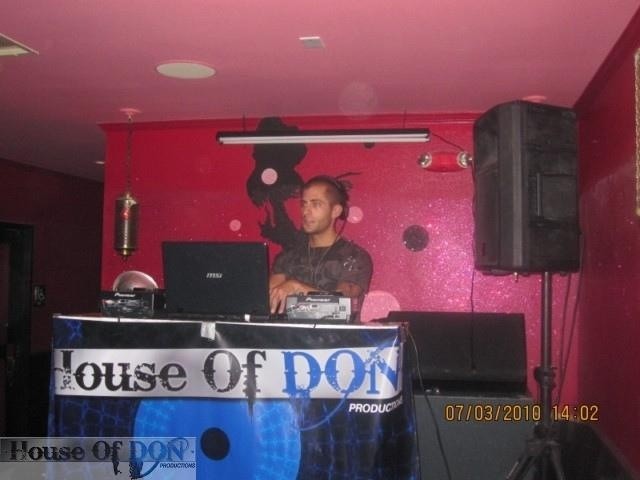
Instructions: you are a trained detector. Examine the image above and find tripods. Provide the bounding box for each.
[506,271,577,480]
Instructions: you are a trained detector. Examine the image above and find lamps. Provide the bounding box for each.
[113,108,142,262]
[216,110,430,145]
[416,150,472,171]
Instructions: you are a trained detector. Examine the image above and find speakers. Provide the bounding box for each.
[386,309,528,397]
[473,99,581,277]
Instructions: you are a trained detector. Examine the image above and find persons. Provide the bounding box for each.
[268,173,374,323]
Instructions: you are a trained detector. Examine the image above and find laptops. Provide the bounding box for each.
[161,240,287,323]
